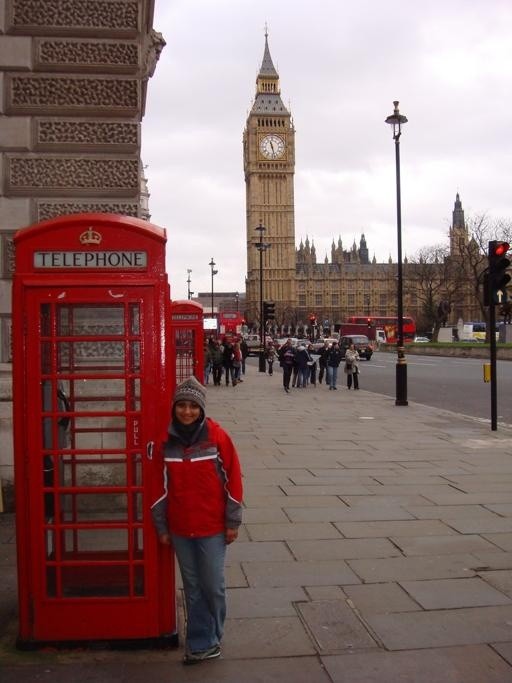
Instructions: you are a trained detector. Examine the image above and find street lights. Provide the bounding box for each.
[385,99,408,405]
[235,290,239,312]
[251,224,272,371]
[366,294,370,339]
[208,257,218,318]
[186,267,195,299]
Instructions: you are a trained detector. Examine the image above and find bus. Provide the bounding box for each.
[454,318,512,342]
[340,315,416,343]
[176,311,245,358]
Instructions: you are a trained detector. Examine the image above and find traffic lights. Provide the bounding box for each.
[310,312,315,322]
[368,318,371,329]
[485,239,511,307]
[263,300,275,320]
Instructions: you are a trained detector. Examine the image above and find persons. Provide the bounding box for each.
[203,332,361,393]
[151,375,244,665]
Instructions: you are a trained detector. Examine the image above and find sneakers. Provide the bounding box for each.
[184,649,220,663]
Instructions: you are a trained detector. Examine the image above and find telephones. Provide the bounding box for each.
[58,390,70,426]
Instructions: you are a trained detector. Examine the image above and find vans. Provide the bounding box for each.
[423,331,455,342]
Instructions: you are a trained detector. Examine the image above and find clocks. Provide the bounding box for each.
[258,131,288,160]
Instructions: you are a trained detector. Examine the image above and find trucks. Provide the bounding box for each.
[332,323,386,352]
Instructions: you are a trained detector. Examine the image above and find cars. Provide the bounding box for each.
[413,336,430,343]
[271,331,340,356]
[336,334,373,360]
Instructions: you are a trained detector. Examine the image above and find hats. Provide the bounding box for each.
[175,377,206,408]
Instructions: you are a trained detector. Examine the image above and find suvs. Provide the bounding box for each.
[243,334,275,355]
